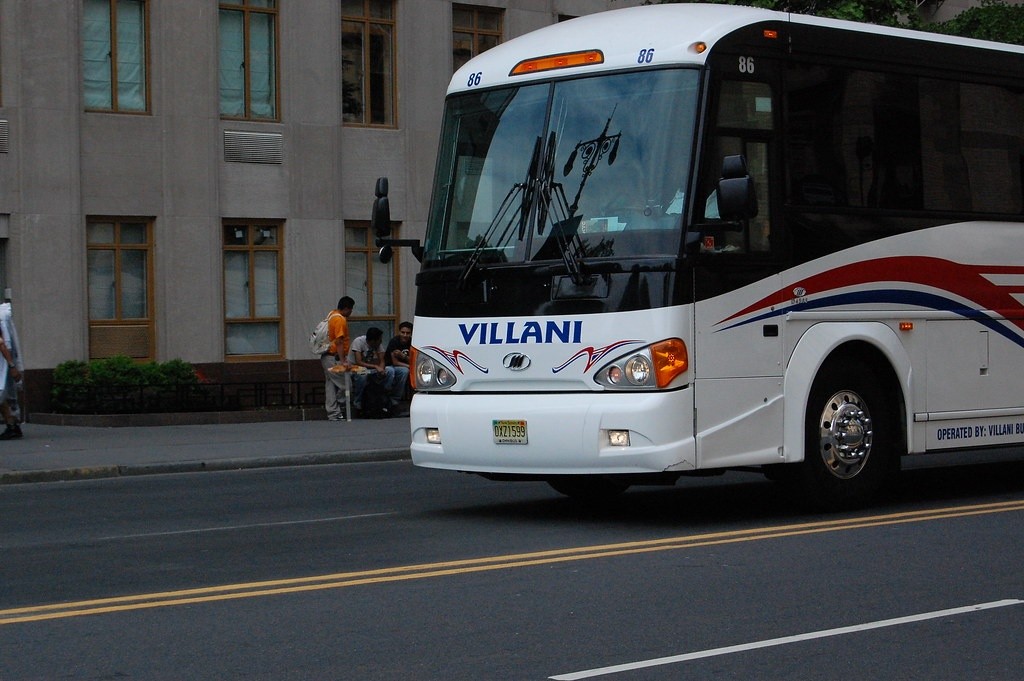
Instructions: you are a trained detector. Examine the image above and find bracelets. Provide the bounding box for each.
[8,363,16,367]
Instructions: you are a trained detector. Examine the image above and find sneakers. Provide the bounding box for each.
[0,424,22,440]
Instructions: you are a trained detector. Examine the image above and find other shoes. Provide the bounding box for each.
[331,413,346,420]
[357,410,368,418]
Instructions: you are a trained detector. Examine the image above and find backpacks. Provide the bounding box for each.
[310,310,343,355]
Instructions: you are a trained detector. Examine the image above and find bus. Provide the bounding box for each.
[373,3,1024,511]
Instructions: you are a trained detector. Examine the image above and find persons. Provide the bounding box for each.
[662,189,726,250]
[0,303,24,441]
[384,321,413,409]
[321,296,355,420]
[349,327,394,416]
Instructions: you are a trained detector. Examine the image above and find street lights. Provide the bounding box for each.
[527,101,622,259]
